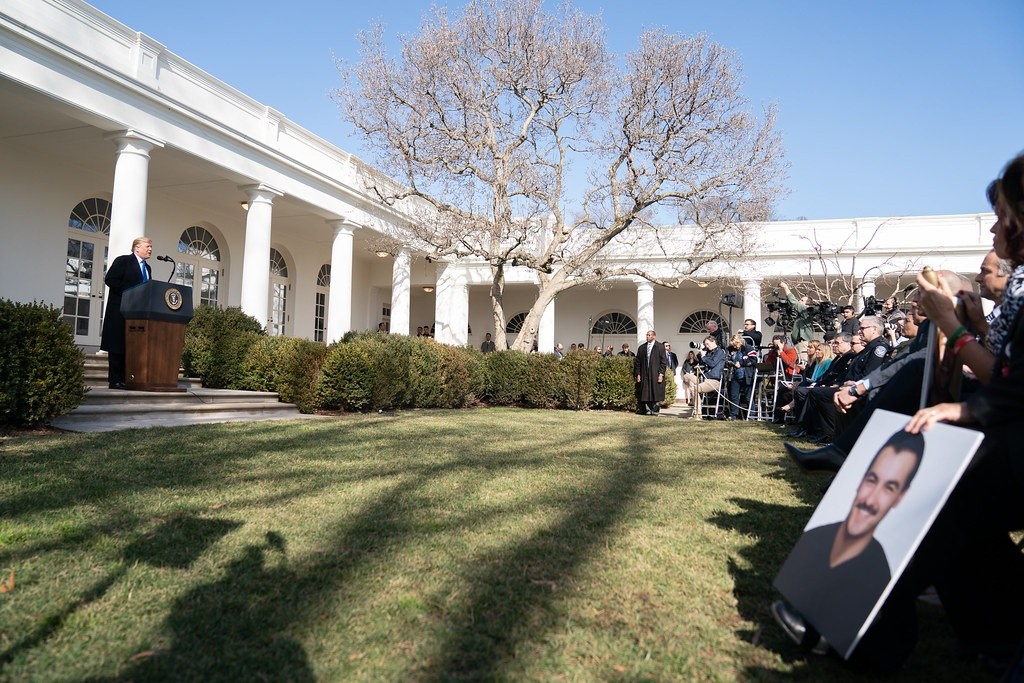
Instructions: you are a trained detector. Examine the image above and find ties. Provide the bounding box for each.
[140,260,147,282]
[646,344,651,363]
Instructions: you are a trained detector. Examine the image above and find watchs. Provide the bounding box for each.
[849,385,860,398]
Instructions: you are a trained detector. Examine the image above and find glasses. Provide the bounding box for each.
[859,326,876,331]
[745,324,753,326]
[850,341,862,345]
[834,340,842,345]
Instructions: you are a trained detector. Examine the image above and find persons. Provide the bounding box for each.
[99,237,152,389]
[417,326,433,338]
[683,154,1024,683]
[774,429,926,655]
[662,342,678,375]
[377,322,386,332]
[481,333,495,354]
[635,331,666,416]
[554,342,634,357]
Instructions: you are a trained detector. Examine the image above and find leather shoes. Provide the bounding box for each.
[770,600,834,656]
[109,383,125,389]
[789,429,829,442]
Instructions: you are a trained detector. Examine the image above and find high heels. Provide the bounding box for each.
[784,443,848,495]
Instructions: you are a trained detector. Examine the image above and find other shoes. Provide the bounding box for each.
[778,424,789,429]
[772,419,782,423]
[777,405,789,413]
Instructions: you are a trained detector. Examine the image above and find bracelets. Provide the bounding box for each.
[946,325,966,349]
[953,335,976,355]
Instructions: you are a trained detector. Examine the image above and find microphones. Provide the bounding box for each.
[765,300,779,304]
[156,256,173,262]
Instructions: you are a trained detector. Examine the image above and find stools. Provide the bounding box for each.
[701,370,729,416]
[783,374,803,421]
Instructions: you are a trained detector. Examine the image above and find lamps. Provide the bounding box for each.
[426,257,431,263]
[376,251,389,258]
[423,287,434,292]
[241,201,249,211]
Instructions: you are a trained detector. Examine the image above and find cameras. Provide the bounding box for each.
[689,341,711,352]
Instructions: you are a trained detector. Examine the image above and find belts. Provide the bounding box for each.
[707,378,720,381]
